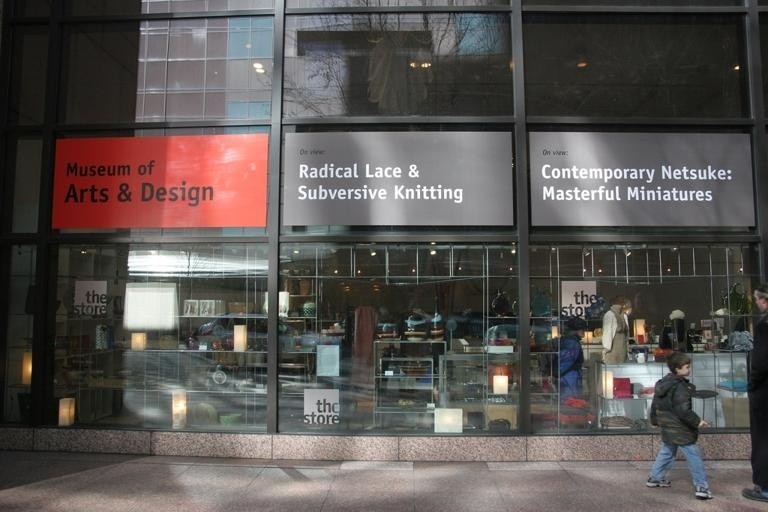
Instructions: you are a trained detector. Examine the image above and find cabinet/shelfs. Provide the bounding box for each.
[8,272,753,432]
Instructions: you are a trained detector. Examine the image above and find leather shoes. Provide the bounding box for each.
[742,485,768,502]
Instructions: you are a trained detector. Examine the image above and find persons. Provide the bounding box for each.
[547,316,590,416]
[645,352,713,498]
[686,322,702,353]
[741,284,768,503]
[487,292,518,348]
[602,304,631,365]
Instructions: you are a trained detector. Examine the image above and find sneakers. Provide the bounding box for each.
[647,475,672,487]
[695,485,713,499]
